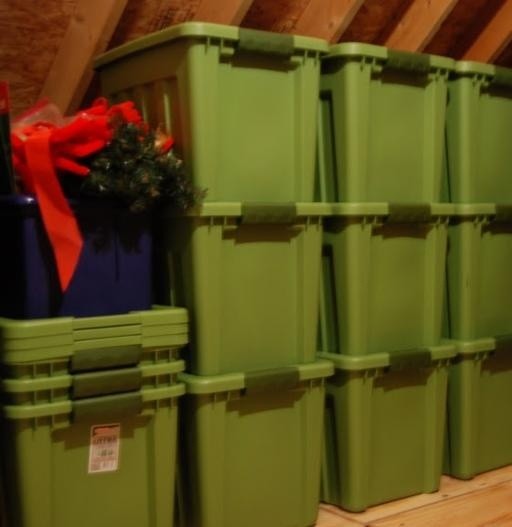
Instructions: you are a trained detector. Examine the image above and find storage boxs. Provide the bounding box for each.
[177,359,337,527]
[315,203,456,356]
[440,202,511,339]
[439,60,512,202]
[313,43,457,203]
[153,203,332,378]
[318,344,457,514]
[441,336,512,481]
[91,22,329,203]
